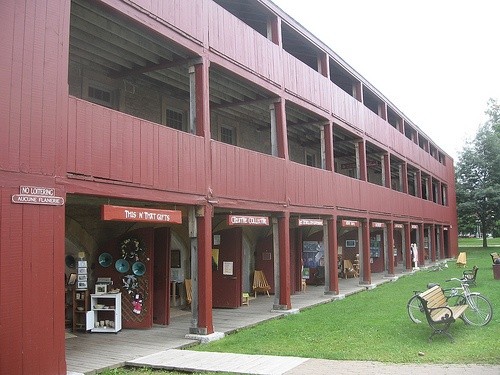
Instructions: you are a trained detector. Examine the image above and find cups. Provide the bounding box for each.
[95,320,114,330]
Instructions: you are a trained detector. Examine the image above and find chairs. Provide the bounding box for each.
[253,270,271,298]
[344,260,357,279]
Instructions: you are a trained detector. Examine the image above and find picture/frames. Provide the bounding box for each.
[303,241,318,253]
[95,284,107,294]
[171,249,181,268]
[346,240,356,247]
[262,252,271,261]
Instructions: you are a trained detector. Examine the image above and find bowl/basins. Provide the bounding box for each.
[110,306,115,309]
[93,304,105,309]
[76,306,85,311]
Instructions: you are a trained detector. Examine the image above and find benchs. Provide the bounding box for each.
[489,252,500,264]
[463,264,479,287]
[417,284,469,343]
[456,252,466,268]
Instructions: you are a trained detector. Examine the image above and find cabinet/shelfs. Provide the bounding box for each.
[86,293,122,334]
[73,285,90,333]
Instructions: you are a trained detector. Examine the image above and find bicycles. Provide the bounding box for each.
[406,277,493,327]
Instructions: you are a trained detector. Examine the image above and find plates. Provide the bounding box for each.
[104,307,109,309]
[99,253,113,267]
[114,259,130,273]
[132,262,147,276]
